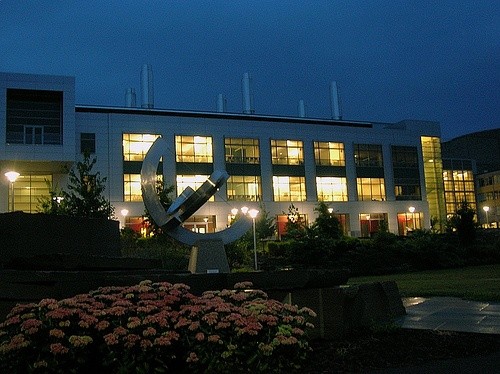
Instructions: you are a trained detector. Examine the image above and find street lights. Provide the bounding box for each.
[409,207,415,230]
[5,171,20,212]
[249,209,259,271]
[121,209,128,227]
[483,206,489,228]
